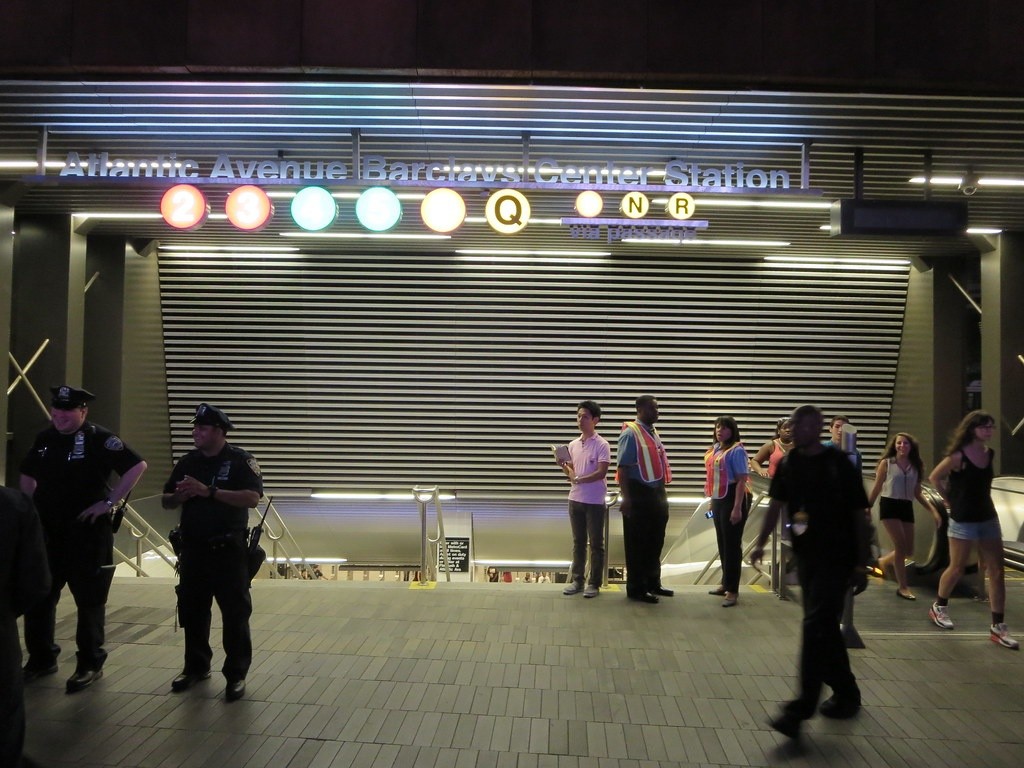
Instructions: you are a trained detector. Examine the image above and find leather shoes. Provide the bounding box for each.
[171,665,211,689]
[650,585,674,596]
[225,679,246,701]
[628,590,659,603]
[66,666,104,691]
[21,660,58,684]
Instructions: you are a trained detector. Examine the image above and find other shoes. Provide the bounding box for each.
[896,588,916,600]
[818,687,862,717]
[771,712,802,740]
[583,585,599,597]
[878,557,890,577]
[563,581,584,595]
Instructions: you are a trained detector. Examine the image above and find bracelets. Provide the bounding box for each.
[938,487,944,493]
[574,476,579,484]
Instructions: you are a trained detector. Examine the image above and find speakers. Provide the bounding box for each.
[909,257,932,273]
[137,239,160,257]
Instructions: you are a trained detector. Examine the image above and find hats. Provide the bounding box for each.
[189,402,237,432]
[49,384,95,410]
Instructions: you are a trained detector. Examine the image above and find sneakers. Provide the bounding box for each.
[990,622,1019,648]
[929,601,954,629]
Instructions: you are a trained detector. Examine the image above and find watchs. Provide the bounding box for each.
[207,484,218,499]
[104,497,113,507]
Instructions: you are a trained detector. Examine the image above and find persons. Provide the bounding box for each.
[928,410,1020,648]
[554,402,611,598]
[750,420,795,479]
[867,432,942,600]
[0,485,52,768]
[299,565,328,580]
[704,416,753,607]
[521,571,551,584]
[19,386,147,695]
[821,414,862,477]
[616,395,674,604]
[749,406,875,740]
[161,403,264,701]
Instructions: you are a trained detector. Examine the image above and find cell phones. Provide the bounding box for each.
[705,509,713,519]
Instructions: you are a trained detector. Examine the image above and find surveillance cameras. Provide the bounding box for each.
[957,174,982,196]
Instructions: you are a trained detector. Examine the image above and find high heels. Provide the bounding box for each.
[722,591,739,607]
[708,583,727,594]
[747,549,765,566]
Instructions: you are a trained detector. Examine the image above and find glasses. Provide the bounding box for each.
[979,426,997,432]
